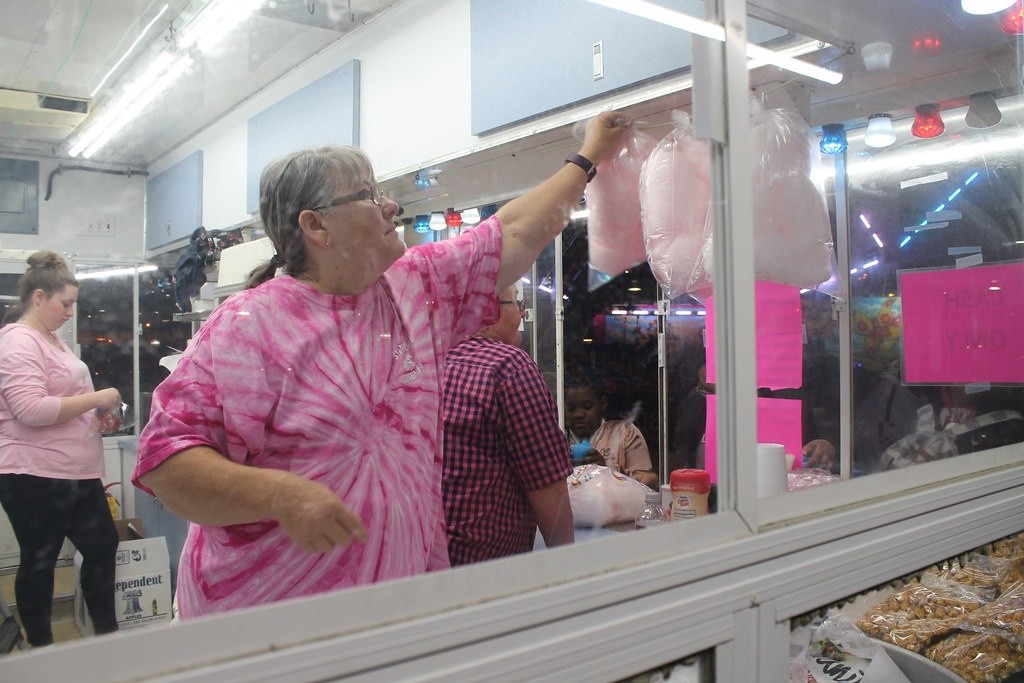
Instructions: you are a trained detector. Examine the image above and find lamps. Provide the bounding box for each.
[820,0,1024,154]
[55,2,264,159]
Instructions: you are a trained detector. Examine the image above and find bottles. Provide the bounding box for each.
[670,469,711,522]
[635,492,670,530]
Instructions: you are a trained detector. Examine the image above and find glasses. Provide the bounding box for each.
[499,297,526,312]
[311,183,384,210]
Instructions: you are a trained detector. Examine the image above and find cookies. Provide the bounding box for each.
[858,537,1024,683]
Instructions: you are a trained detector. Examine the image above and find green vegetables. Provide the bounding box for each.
[810,640,848,660]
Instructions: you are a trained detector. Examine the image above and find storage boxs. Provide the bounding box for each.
[73,518,172,638]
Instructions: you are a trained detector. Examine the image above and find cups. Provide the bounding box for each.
[571,445,592,460]
[756,442,788,500]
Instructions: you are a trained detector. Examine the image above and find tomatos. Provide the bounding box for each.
[98,412,120,433]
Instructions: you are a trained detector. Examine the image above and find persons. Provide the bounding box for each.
[131,111,632,616]
[441,282,575,568]
[696,355,840,474]
[0,250,123,647]
[568,367,659,492]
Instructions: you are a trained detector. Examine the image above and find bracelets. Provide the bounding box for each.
[564,152,597,183]
